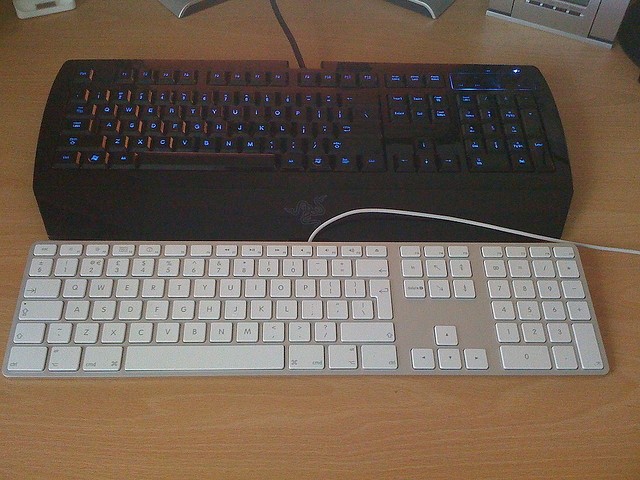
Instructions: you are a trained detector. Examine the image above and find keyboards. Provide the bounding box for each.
[2,242,609,378]
[32,60,573,242]
